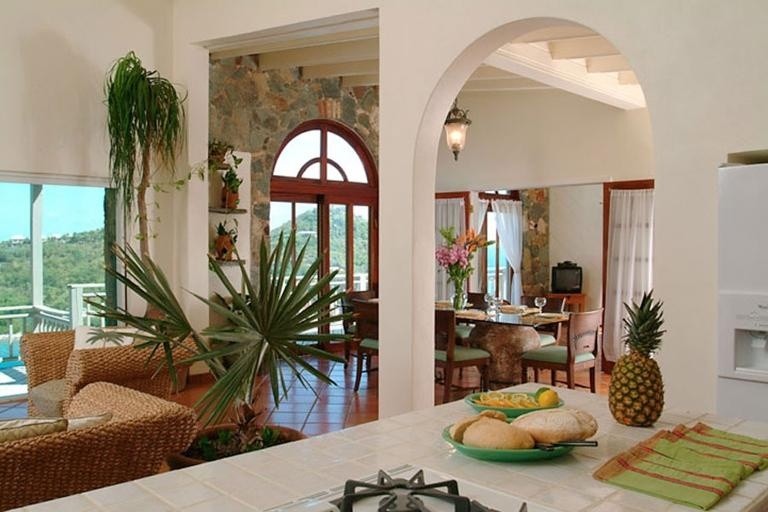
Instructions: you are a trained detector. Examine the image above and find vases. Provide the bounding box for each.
[448,272,469,314]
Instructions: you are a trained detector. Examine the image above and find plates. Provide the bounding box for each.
[465,390,565,420]
[442,421,576,464]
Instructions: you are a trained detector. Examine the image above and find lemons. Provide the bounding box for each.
[474,390,559,408]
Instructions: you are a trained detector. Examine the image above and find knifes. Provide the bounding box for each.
[536,438,598,453]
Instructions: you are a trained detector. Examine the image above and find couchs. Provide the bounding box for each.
[18,325,198,423]
[2,383,196,512]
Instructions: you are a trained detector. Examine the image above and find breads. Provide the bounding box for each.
[449,407,599,450]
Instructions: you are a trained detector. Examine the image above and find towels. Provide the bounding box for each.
[597,440,743,509]
[670,424,768,472]
[691,423,768,449]
[636,431,761,485]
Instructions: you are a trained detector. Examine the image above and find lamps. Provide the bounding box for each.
[444,109,473,161]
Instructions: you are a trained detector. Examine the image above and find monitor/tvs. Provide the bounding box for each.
[551,266,582,294]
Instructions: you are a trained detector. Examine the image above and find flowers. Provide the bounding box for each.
[438,229,483,268]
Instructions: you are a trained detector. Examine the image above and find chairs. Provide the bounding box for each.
[351,297,381,393]
[434,312,491,403]
[521,309,604,393]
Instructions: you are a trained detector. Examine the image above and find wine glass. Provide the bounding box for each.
[484,295,503,322]
[534,297,547,316]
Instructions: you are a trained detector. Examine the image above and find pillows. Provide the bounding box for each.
[0,418,69,441]
[68,412,113,430]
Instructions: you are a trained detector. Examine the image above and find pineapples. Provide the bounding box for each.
[608,288,666,427]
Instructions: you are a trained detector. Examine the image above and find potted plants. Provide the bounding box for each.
[214,222,235,259]
[221,165,244,210]
[85,232,356,467]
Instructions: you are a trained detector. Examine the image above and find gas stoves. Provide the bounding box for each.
[255,461,558,512]
